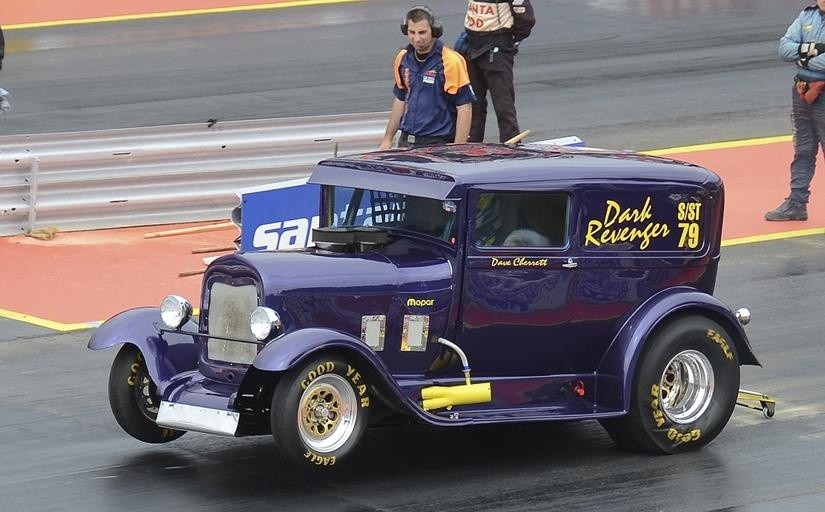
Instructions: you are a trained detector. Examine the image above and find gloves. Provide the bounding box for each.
[796,41,824,68]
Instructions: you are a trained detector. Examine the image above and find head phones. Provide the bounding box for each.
[401,5,443,38]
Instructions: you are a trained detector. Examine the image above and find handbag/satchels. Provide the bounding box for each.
[453,30,469,61]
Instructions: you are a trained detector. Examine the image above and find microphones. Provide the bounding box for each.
[416,37,437,47]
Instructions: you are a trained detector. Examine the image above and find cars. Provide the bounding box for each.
[83,142,764,477]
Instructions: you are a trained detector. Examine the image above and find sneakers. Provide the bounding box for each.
[765,199,808,223]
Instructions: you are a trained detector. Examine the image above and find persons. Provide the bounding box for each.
[474,190,505,248]
[765,1,824,222]
[377,4,478,149]
[463,0,536,145]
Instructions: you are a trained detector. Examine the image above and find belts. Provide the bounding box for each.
[401,131,454,144]
